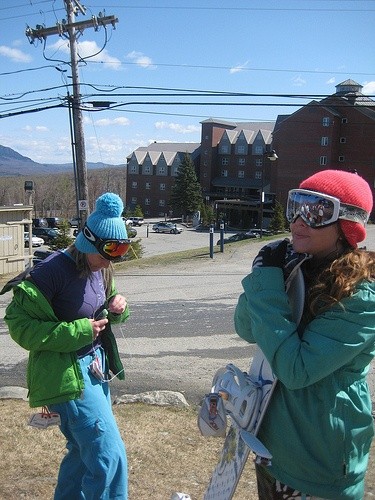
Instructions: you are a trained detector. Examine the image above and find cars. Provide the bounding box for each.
[69,217,82,227]
[217,233,258,247]
[248,229,280,241]
[24,232,44,248]
[123,228,137,239]
[152,221,183,234]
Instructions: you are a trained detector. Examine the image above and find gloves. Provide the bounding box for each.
[251,237,309,294]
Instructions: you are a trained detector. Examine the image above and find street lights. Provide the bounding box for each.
[259,150,278,239]
[65,99,117,237]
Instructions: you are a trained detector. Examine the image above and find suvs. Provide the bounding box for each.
[32,219,49,231]
[35,229,65,245]
[121,217,134,227]
[129,217,143,226]
[44,218,63,230]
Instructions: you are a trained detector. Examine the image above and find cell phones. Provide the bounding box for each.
[95,309,108,321]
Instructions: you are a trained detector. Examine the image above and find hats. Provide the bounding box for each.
[299,169,373,251]
[74,192,128,255]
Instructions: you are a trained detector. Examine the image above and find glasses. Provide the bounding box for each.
[81,225,132,260]
[286,188,369,229]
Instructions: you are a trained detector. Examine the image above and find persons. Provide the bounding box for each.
[1,193,130,500]
[232,169,375,500]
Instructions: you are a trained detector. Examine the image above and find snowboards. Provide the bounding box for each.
[192,266,307,500]
